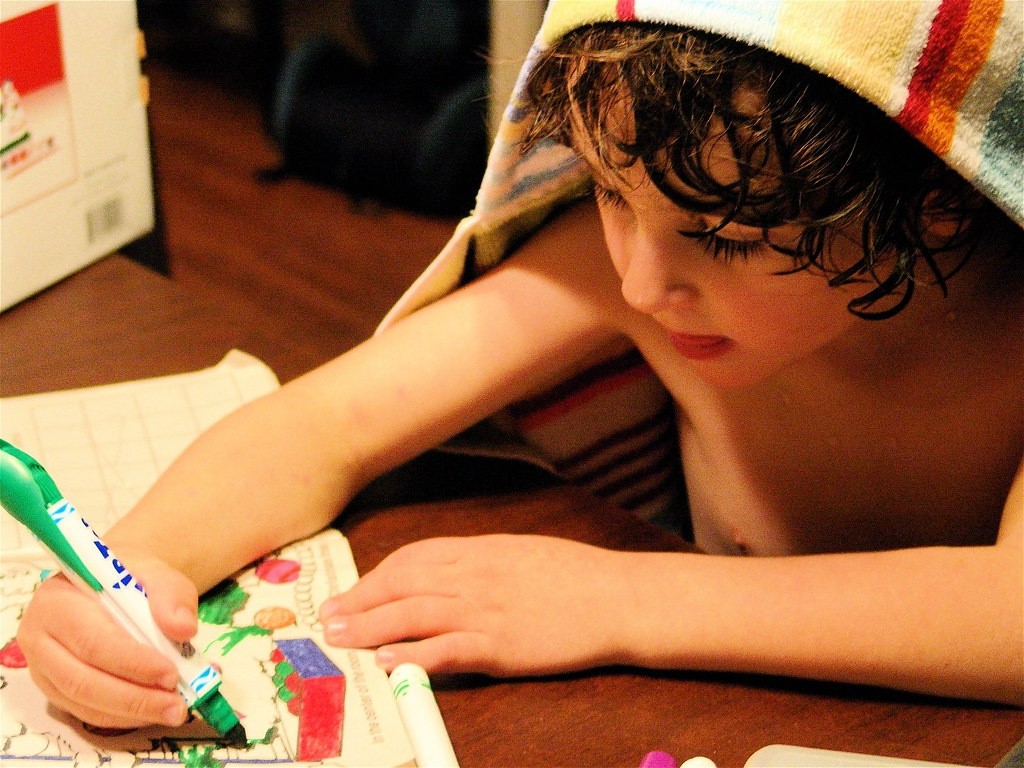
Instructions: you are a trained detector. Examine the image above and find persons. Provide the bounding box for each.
[17,0,1024,730]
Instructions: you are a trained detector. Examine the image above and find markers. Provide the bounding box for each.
[0,440,250,749]
